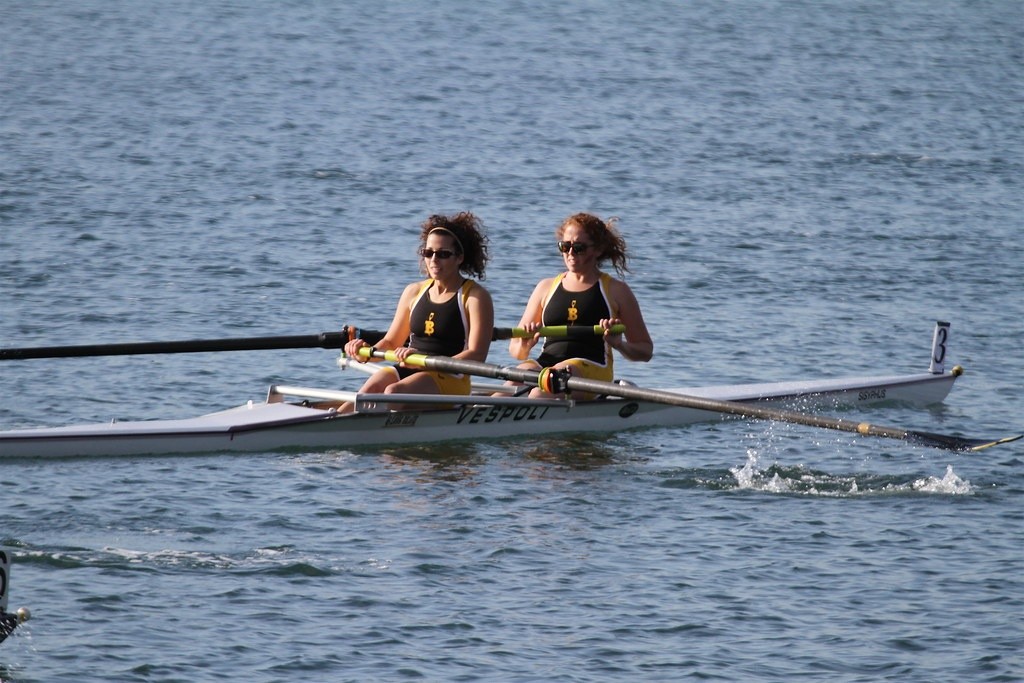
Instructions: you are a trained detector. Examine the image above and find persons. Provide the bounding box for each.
[336,211,494,413]
[490,213,654,403]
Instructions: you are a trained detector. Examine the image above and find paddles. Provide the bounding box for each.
[0,322,625,363]
[350,339,1024,455]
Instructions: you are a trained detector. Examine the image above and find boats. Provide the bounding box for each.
[0,364,964,458]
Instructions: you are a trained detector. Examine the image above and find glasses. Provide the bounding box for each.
[421,249,458,259]
[558,240,599,254]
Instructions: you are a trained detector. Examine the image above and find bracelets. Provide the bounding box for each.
[357,357,370,364]
[613,342,621,349]
